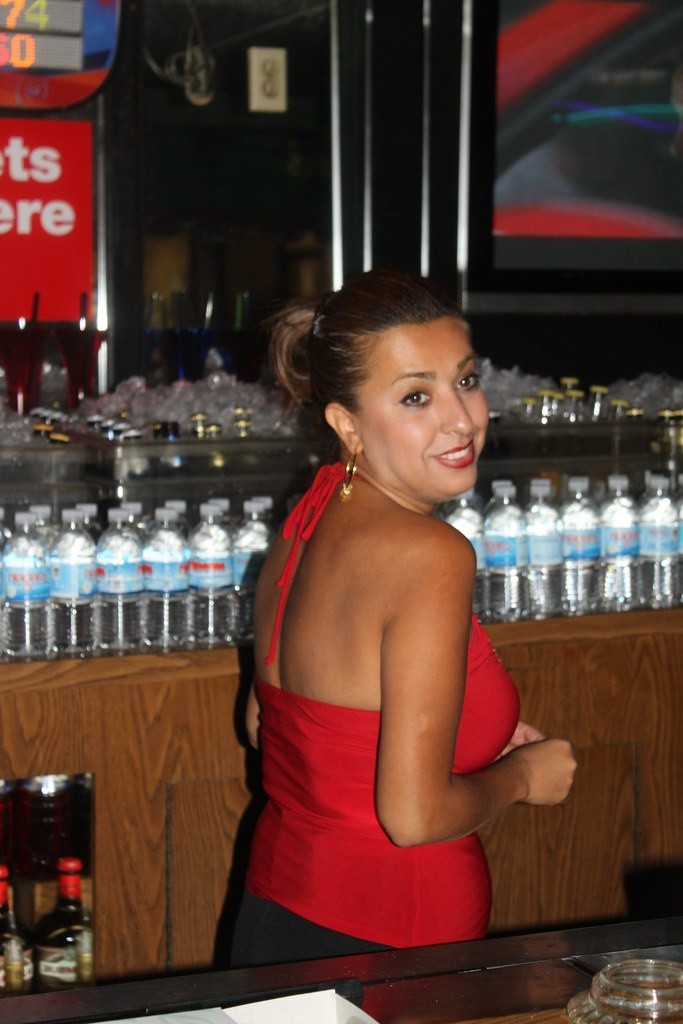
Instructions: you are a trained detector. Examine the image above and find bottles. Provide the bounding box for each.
[518,373,646,425]
[654,409,678,471]
[674,409,683,471]
[0,472,683,657]
[0,859,94,994]
[29,406,260,479]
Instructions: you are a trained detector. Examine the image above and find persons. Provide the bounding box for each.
[229,269,577,970]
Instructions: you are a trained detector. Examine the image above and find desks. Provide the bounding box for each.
[0,914,683,1024]
